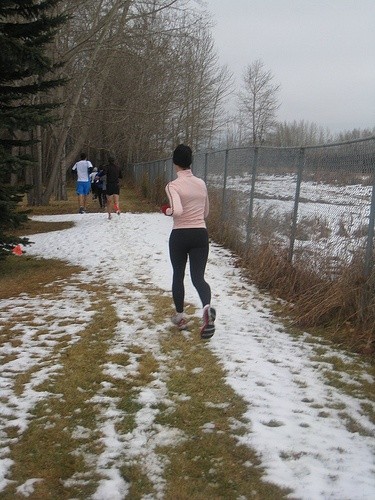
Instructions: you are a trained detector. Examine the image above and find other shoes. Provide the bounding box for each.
[200,304,217,338]
[175,317,187,330]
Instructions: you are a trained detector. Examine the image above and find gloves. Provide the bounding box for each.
[162,203,170,214]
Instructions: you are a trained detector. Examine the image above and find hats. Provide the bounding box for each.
[172,144,192,167]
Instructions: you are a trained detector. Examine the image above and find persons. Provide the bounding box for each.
[96,156,125,220]
[71,153,94,214]
[88,166,108,210]
[161,143,217,339]
